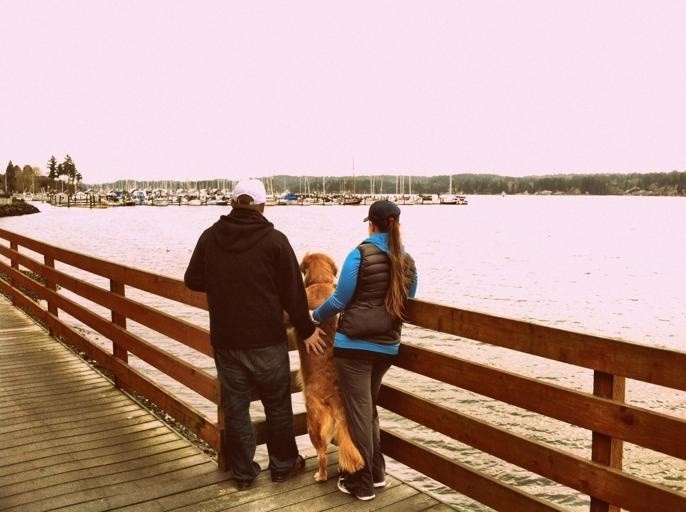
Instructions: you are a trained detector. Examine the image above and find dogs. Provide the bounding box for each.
[283,253,366,482]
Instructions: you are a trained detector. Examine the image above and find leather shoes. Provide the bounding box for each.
[231,462,261,490]
[269,455,305,483]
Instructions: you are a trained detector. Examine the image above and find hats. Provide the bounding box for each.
[361,200,401,222]
[230,180,267,205]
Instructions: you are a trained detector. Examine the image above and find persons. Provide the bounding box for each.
[307,200,417,501]
[184,178,328,491]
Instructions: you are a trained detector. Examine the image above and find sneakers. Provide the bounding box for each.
[337,462,387,501]
